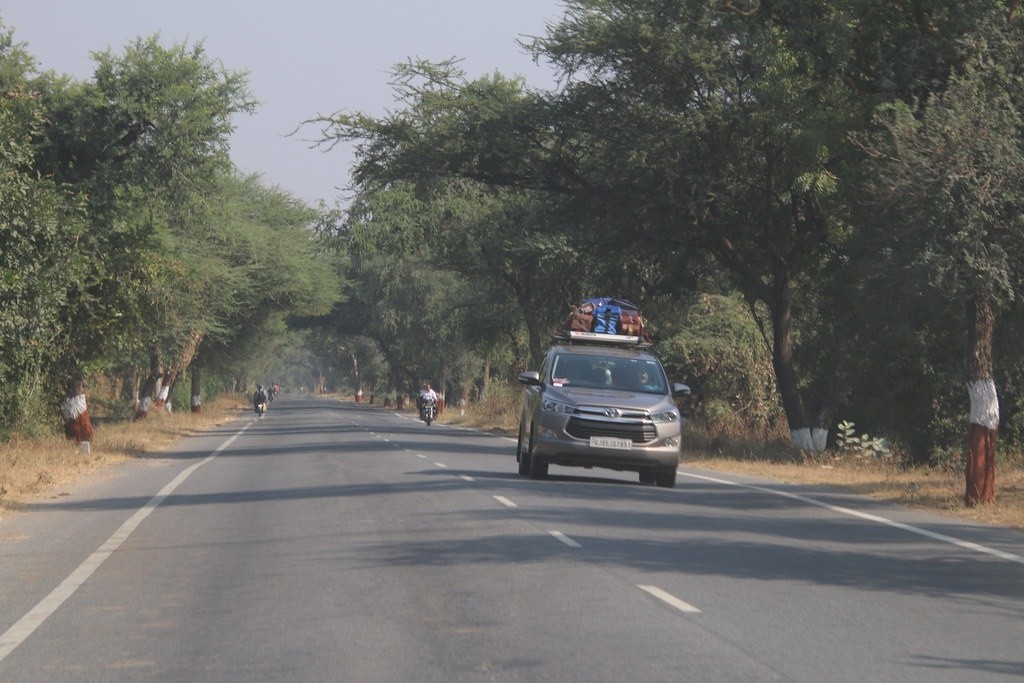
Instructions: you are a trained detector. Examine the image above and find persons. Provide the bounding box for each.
[416,380,439,420]
[267,383,280,400]
[253,382,268,413]
[630,369,658,391]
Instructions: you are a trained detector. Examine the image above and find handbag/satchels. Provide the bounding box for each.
[593,305,620,335]
[620,315,643,336]
[567,305,593,331]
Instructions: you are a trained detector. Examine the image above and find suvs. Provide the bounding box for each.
[515,331,692,488]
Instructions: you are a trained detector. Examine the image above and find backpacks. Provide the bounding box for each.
[257,389,266,403]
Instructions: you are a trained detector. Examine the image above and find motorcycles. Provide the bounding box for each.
[256,400,267,417]
[420,397,437,426]
